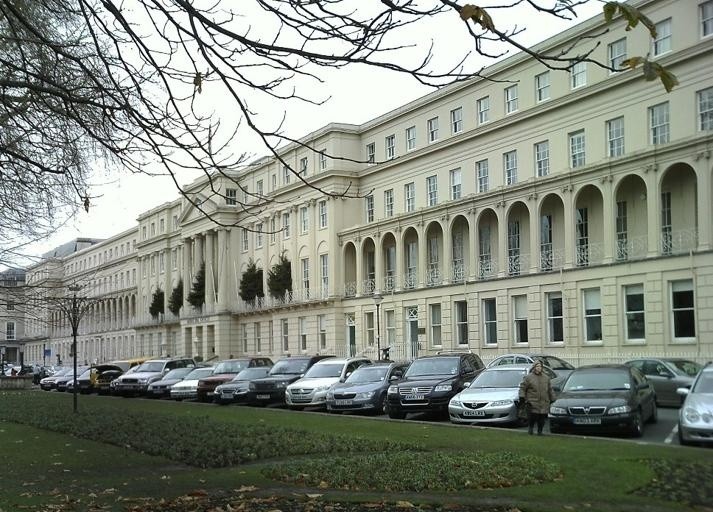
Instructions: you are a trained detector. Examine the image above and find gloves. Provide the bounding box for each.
[519,396,525,405]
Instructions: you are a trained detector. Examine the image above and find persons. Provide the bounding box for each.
[33,363,42,386]
[3,364,9,371]
[40,363,46,385]
[11,368,17,376]
[519,360,556,434]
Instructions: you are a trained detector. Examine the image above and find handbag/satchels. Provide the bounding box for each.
[514,401,532,421]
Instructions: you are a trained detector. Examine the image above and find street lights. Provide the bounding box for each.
[65,277,83,412]
[367,293,387,361]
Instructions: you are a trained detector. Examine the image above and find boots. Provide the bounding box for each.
[538,432,544,436]
[528,427,534,435]
